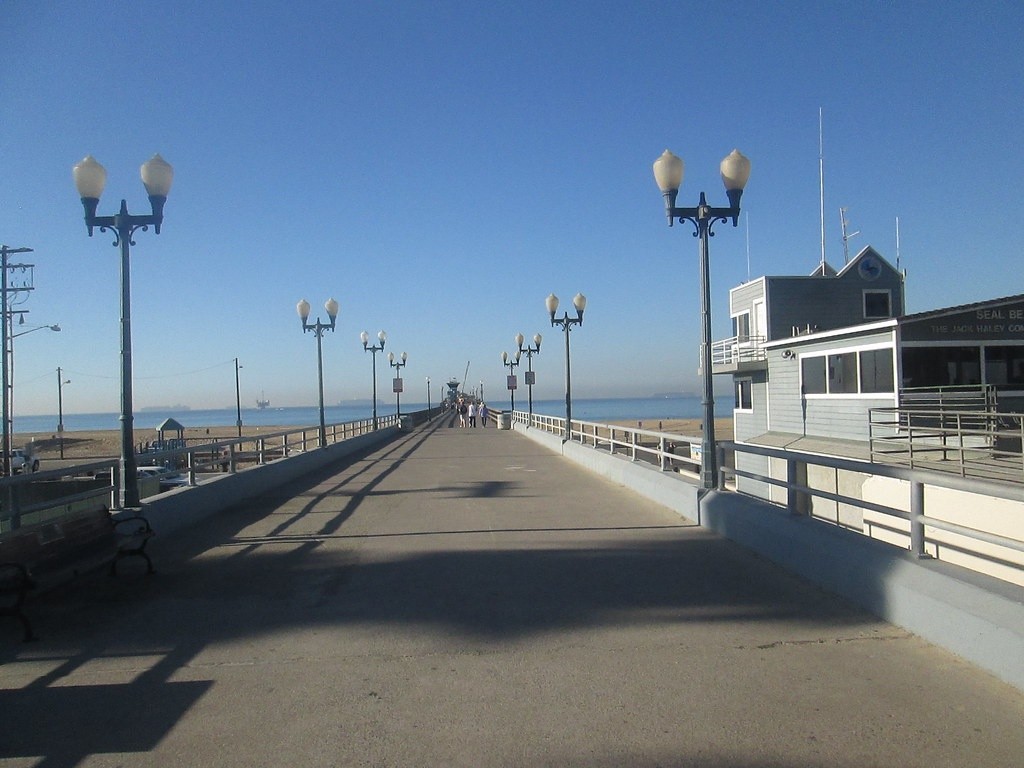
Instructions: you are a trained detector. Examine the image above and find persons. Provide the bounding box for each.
[657,442,661,463]
[454,399,467,427]
[468,402,478,428]
[667,442,676,465]
[479,402,488,427]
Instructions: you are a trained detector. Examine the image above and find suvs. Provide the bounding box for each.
[0,449,40,472]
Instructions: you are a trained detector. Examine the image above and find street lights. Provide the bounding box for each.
[297,297,338,447]
[70,152,173,582]
[501,351,521,411]
[58,380,71,459]
[480,380,484,402]
[388,351,407,418]
[4,324,61,476]
[652,148,752,489]
[515,332,542,412]
[545,292,586,438]
[426,376,431,421]
[360,329,387,431]
